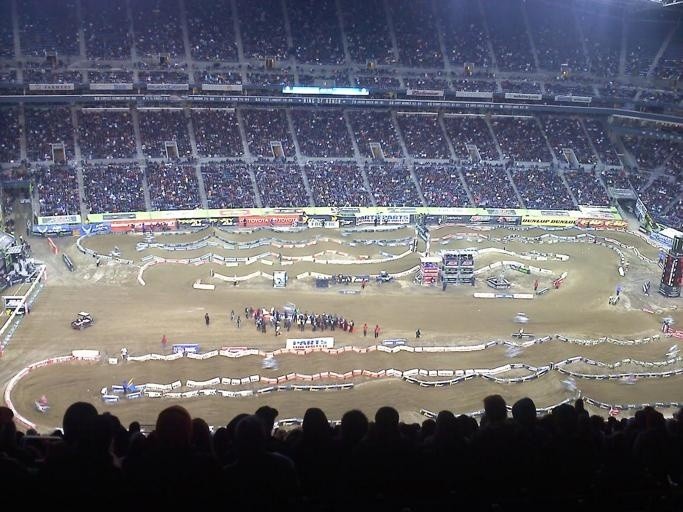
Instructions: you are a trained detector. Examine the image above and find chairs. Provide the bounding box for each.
[20,435,64,459]
